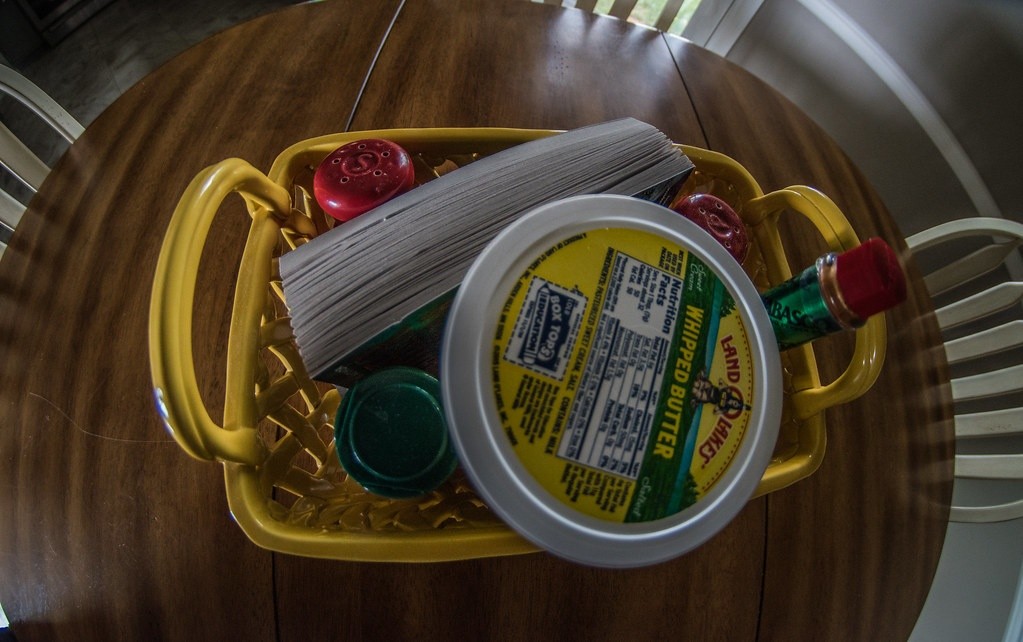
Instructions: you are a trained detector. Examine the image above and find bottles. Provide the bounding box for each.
[335,369,456,499]
[319,140,441,328]
[768,237,908,354]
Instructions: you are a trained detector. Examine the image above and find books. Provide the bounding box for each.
[277,115,696,388]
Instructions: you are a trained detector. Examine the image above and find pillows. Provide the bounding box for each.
[521,0,766,58]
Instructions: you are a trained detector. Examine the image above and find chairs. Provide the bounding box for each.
[0,64,88,264]
[895,215,1023,525]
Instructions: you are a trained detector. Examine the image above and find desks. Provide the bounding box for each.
[0,0,956,640]
